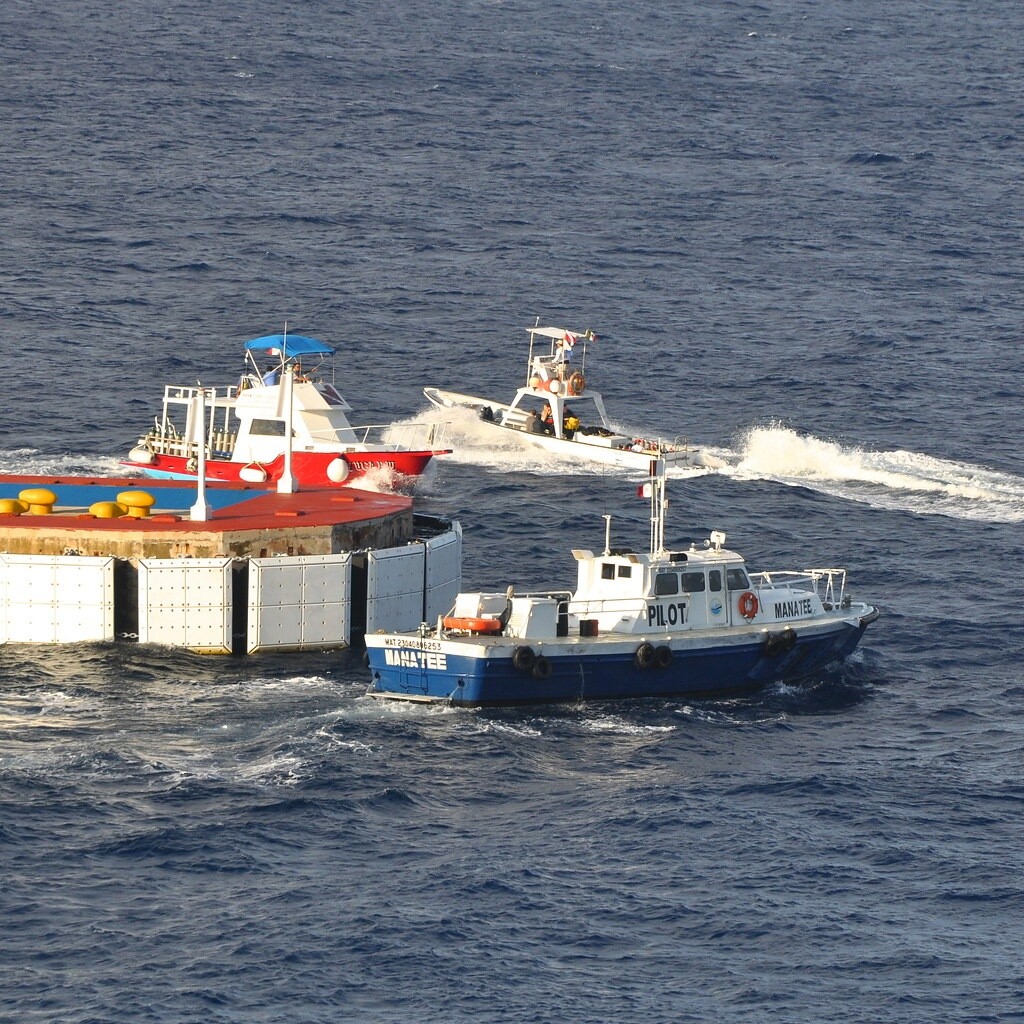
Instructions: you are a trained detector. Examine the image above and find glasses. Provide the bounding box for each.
[296,366,299,368]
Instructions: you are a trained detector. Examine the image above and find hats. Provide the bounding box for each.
[556,340,563,344]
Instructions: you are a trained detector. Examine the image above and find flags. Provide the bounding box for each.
[587,330,597,343]
[565,329,576,347]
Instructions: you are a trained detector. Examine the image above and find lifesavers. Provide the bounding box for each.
[533,656,553,680]
[570,372,585,394]
[739,593,759,618]
[513,646,534,672]
[634,642,655,668]
[655,645,674,669]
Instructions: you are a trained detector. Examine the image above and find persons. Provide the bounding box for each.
[526,402,578,439]
[552,339,570,382]
[292,362,318,383]
[263,365,282,386]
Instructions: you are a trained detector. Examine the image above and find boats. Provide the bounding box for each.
[363,473,882,711]
[129,333,454,492]
[424,316,701,470]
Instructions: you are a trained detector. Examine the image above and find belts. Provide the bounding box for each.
[559,362,566,364]
